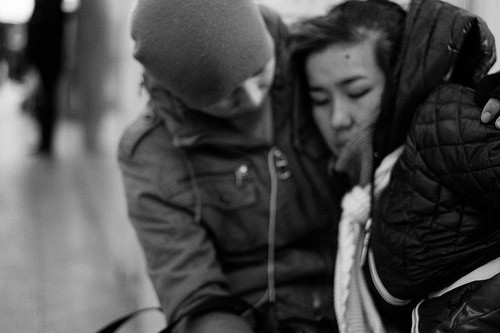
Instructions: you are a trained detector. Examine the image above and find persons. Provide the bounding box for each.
[284,0,500,333]
[9,2,65,154]
[117,0,500,333]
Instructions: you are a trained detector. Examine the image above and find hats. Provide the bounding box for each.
[131,1,275,111]
